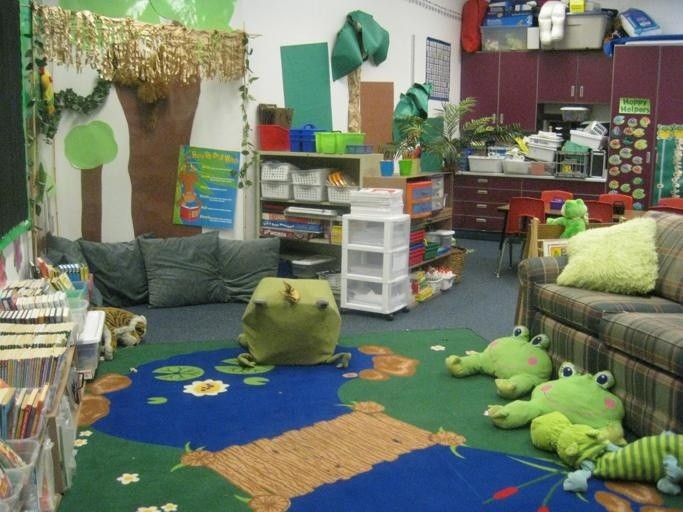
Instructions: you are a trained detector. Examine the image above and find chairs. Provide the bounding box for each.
[495,189,682,279]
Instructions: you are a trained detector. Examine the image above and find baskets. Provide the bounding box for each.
[345,143,376,155]
[289,123,331,153]
[327,185,360,204]
[315,130,368,155]
[288,167,341,186]
[292,184,328,204]
[407,227,455,266]
[260,181,294,201]
[260,161,301,181]
[408,269,444,303]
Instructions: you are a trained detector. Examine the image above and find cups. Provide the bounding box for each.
[378,159,421,176]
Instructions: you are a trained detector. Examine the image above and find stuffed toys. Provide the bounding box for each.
[484,362,628,446]
[90,306,147,361]
[444,325,551,399]
[529,412,683,496]
[544,199,588,241]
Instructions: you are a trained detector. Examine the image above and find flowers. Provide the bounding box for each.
[396,130,420,160]
[429,342,446,352]
[74,428,179,511]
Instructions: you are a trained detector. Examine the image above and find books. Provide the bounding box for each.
[0,254,90,512]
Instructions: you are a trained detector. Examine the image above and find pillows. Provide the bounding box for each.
[555,216,660,297]
[44,234,104,306]
[81,242,147,311]
[211,234,283,307]
[136,230,231,308]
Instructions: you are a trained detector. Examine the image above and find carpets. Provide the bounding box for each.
[55,324,682,511]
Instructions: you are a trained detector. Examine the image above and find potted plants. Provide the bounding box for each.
[376,143,395,176]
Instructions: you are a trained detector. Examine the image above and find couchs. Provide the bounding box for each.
[513,209,682,439]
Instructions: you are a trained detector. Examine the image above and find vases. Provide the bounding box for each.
[398,160,412,177]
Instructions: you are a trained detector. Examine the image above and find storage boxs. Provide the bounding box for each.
[0,385,55,511]
[539,13,614,51]
[443,106,608,180]
[64,274,95,345]
[288,253,335,279]
[479,26,529,53]
[257,120,374,156]
[72,310,107,374]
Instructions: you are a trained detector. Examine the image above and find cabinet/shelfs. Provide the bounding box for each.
[339,213,410,322]
[253,151,382,309]
[42,273,95,496]
[458,49,537,134]
[522,174,602,200]
[536,51,613,104]
[364,173,453,309]
[606,46,683,210]
[453,171,521,243]
